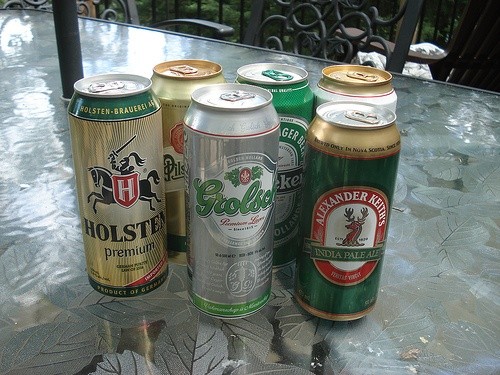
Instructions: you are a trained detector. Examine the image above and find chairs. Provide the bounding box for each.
[333,25,459,79]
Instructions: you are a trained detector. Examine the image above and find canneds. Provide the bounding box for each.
[292,100,402,321]
[184,83,280,319]
[315,64,397,114]
[150,59,224,266]
[233,62,315,268]
[67,73,170,296]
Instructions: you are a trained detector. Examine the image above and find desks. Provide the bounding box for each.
[1,10,498,374]
